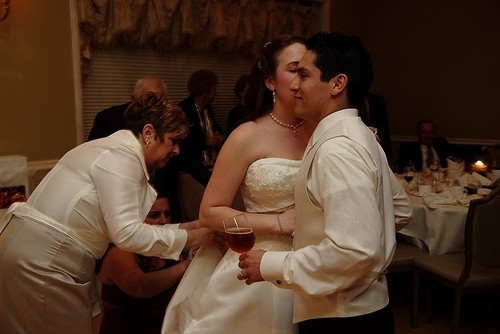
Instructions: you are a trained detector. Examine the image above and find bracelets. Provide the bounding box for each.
[278,212,289,239]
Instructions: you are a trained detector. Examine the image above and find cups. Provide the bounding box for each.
[418,185,431,195]
[452,186,467,197]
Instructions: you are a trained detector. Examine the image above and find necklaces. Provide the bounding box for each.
[269,108,305,137]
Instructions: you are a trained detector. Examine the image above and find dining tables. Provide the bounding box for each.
[395,170,500,256]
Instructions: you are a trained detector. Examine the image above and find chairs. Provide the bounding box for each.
[388,185,500,334]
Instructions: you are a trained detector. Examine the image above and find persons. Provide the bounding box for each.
[0,32,455,334]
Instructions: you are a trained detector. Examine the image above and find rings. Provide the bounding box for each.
[240,271,245,279]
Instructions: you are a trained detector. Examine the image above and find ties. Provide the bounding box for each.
[426,146,435,169]
[200,110,207,152]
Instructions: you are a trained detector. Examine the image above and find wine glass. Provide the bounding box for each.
[402,167,414,196]
[222,213,271,283]
